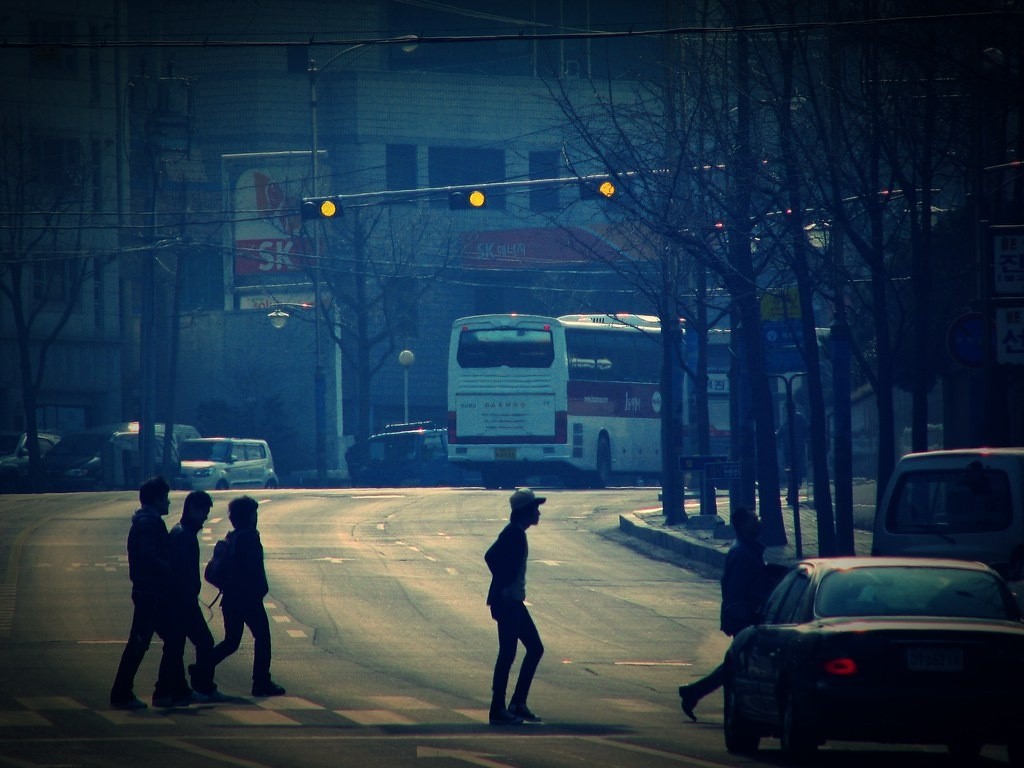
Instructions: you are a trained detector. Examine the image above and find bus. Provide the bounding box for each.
[899,423,944,457]
[681,326,829,486]
[447,311,689,490]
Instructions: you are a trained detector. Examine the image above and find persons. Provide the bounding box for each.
[145,490,230,708]
[775,399,811,506]
[679,508,776,721]
[108,475,198,711]
[483,486,547,726]
[188,496,285,696]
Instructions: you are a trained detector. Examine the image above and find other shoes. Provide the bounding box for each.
[509,702,542,722]
[110,692,147,710]
[191,689,231,704]
[152,695,191,706]
[489,705,522,725]
[188,664,195,689]
[251,681,285,697]
[679,684,698,721]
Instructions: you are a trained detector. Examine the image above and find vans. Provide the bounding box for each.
[873,447,1024,578]
[36,421,204,491]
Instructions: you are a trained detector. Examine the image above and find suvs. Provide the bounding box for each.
[0,429,62,493]
[367,420,447,477]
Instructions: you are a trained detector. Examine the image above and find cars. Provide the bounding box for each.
[721,555,1024,768]
[175,437,279,490]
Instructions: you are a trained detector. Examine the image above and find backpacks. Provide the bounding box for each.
[205,539,229,608]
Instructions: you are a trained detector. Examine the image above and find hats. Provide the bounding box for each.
[732,510,762,539]
[509,488,546,510]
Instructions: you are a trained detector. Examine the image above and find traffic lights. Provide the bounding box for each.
[450,191,487,209]
[579,179,617,200]
[302,200,342,219]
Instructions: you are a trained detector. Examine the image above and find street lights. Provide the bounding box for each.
[398,349,415,424]
[308,29,421,487]
